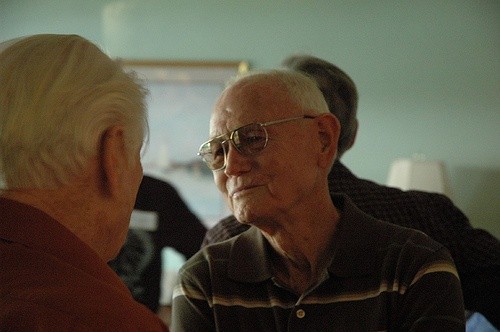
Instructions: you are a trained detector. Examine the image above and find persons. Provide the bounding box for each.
[0,33,171,332]
[168,70,468,332]
[108,176,209,317]
[275,53,500,332]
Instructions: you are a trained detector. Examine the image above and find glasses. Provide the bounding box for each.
[198,115,317,170]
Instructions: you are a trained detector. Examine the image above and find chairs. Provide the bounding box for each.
[109,175,211,313]
